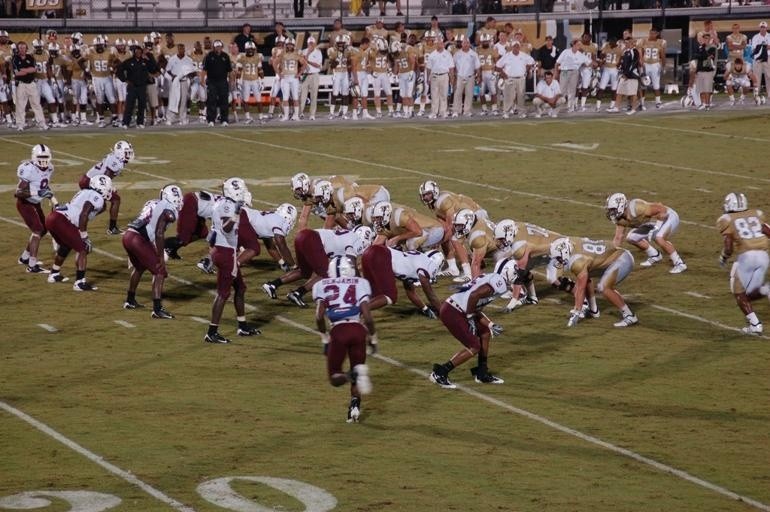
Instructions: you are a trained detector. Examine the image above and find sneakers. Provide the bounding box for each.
[742,322,763,336]
[7,116,188,131]
[639,250,688,273]
[347,364,505,422]
[501,290,639,329]
[200,114,315,128]
[204,282,308,344]
[16,228,216,319]
[329,104,529,121]
[535,96,766,119]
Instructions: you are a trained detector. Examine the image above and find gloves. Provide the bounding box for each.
[718,248,729,267]
[322,304,502,355]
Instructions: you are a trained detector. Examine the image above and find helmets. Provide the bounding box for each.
[606,193,627,225]
[276,173,575,285]
[31,138,252,210]
[275,18,525,48]
[641,74,653,88]
[1,29,257,52]
[722,191,749,213]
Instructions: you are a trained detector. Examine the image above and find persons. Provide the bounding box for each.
[429,257,520,389]
[14,139,135,291]
[606,192,688,274]
[312,256,377,422]
[419,180,639,329]
[2,0,770,129]
[122,172,447,345]
[717,192,770,335]
